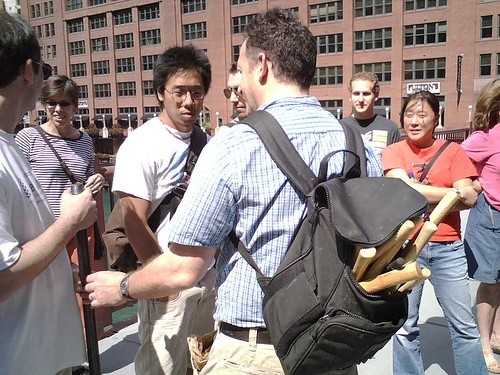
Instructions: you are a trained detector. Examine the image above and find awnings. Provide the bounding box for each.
[140,113,159,120]
[91,115,112,120]
[114,114,137,121]
[34,117,47,122]
[70,116,89,121]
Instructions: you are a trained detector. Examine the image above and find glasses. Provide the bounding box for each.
[164,88,206,100]
[32,60,54,80]
[224,88,243,98]
[44,100,73,106]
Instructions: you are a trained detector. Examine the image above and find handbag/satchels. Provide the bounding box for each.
[101,197,137,273]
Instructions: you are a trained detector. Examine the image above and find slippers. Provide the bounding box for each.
[491,345,500,355]
[483,350,500,374]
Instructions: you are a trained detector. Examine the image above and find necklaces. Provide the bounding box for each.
[158,116,193,139]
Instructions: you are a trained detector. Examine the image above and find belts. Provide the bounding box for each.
[219,321,274,344]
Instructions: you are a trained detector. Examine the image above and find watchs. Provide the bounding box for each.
[119,272,138,304]
[454,187,460,195]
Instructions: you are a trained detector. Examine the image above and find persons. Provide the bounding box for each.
[216,62,256,131]
[84,8,385,375]
[13,71,105,258]
[337,71,401,148]
[380,90,488,375]
[460,77,500,375]
[111,43,216,375]
[0,10,97,375]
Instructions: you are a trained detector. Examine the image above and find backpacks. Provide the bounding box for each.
[227,110,430,375]
